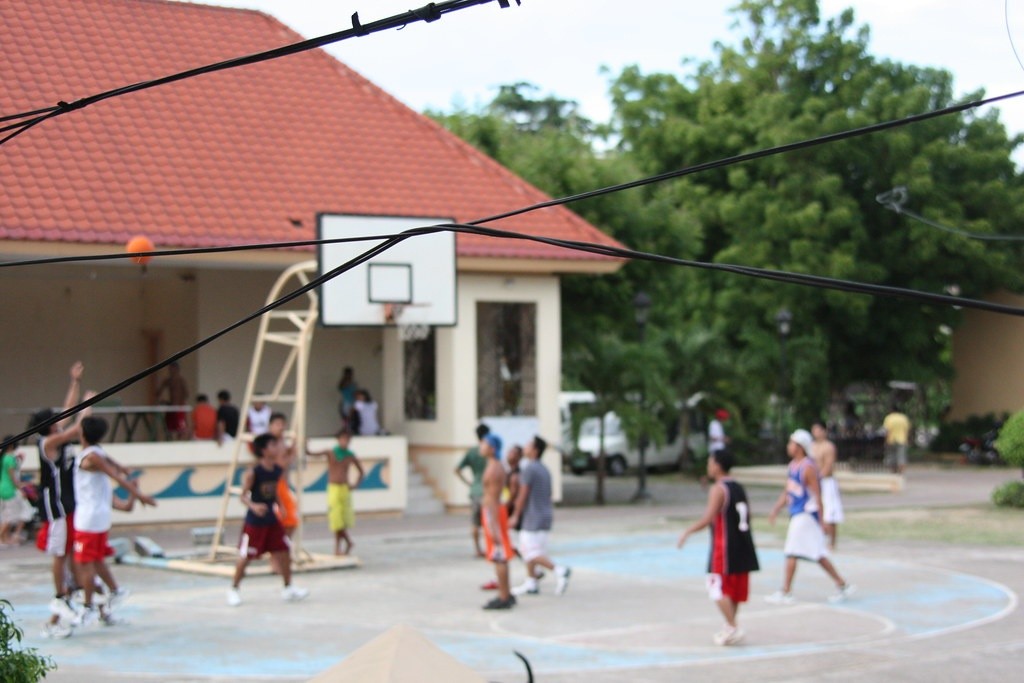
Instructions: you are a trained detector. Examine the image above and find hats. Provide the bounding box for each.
[486,435,502,460]
[716,410,728,421]
[789,429,813,457]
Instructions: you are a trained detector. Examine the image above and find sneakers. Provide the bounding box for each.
[42,584,132,639]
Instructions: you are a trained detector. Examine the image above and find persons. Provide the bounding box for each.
[675,400,912,645]
[455,424,571,613]
[0,364,383,639]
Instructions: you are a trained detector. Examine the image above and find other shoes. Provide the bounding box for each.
[512,581,539,596]
[766,592,795,607]
[535,571,544,579]
[485,594,515,610]
[482,582,498,589]
[829,584,856,603]
[716,628,743,646]
[556,566,570,595]
[227,588,242,606]
[284,586,307,602]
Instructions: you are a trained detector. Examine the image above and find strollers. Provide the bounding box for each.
[960,422,1004,466]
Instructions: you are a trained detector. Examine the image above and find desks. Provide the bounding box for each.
[35,405,193,443]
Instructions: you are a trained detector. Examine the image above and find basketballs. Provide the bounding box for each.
[125,235,155,265]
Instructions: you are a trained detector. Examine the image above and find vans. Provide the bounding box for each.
[563,390,688,477]
